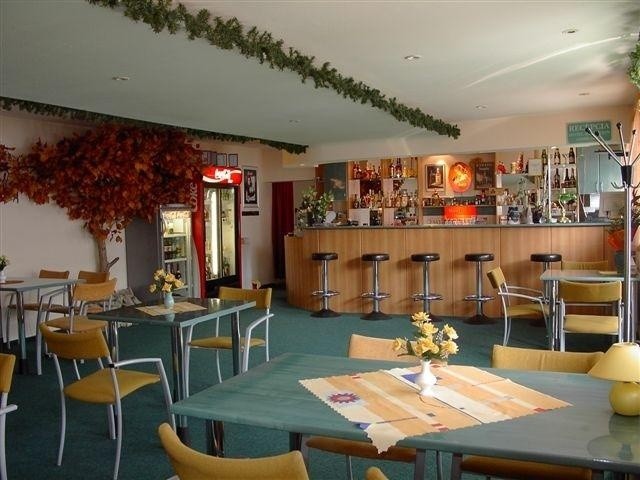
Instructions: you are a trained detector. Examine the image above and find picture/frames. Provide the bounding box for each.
[425,163,445,192]
[474,161,494,189]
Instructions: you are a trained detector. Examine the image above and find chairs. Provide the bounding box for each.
[305,333,448,480]
[34,320,173,479]
[182,285,275,400]
[448,344,607,479]
[558,260,614,271]
[486,267,552,346]
[558,279,622,352]
[364,464,396,480]
[152,416,309,480]
[0,254,118,480]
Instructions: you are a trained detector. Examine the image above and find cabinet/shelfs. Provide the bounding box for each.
[348,160,382,208]
[577,147,626,194]
[383,156,419,206]
[550,147,578,223]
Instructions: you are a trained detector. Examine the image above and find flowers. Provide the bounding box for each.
[391,313,462,364]
[148,267,185,295]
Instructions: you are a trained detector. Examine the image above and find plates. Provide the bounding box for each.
[448,161,473,193]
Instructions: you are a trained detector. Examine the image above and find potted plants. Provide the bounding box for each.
[292,188,335,231]
[604,197,639,275]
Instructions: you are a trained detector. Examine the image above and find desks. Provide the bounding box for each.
[162,351,639,477]
[87,295,259,447]
[539,268,640,348]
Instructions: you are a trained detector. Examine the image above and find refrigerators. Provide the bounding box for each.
[191,165,245,301]
[124,200,193,304]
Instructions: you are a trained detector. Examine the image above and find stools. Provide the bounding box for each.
[310,253,341,318]
[358,253,392,320]
[461,254,497,325]
[530,253,563,320]
[409,253,442,320]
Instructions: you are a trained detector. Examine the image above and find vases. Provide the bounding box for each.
[162,291,176,310]
[415,361,438,403]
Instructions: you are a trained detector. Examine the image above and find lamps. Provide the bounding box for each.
[587,343,639,417]
[586,413,639,465]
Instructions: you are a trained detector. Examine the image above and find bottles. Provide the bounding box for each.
[502,162,504,165]
[541,149,548,166]
[475,160,494,187]
[429,216,476,224]
[405,207,410,221]
[568,200,573,211]
[553,146,576,189]
[499,160,502,165]
[510,161,516,174]
[370,211,382,226]
[352,158,410,178]
[352,189,492,209]
[396,207,403,222]
[510,194,514,204]
[337,211,342,219]
[328,202,333,211]
[203,188,231,282]
[162,236,183,285]
[435,168,442,185]
[517,182,531,207]
[525,162,528,173]
